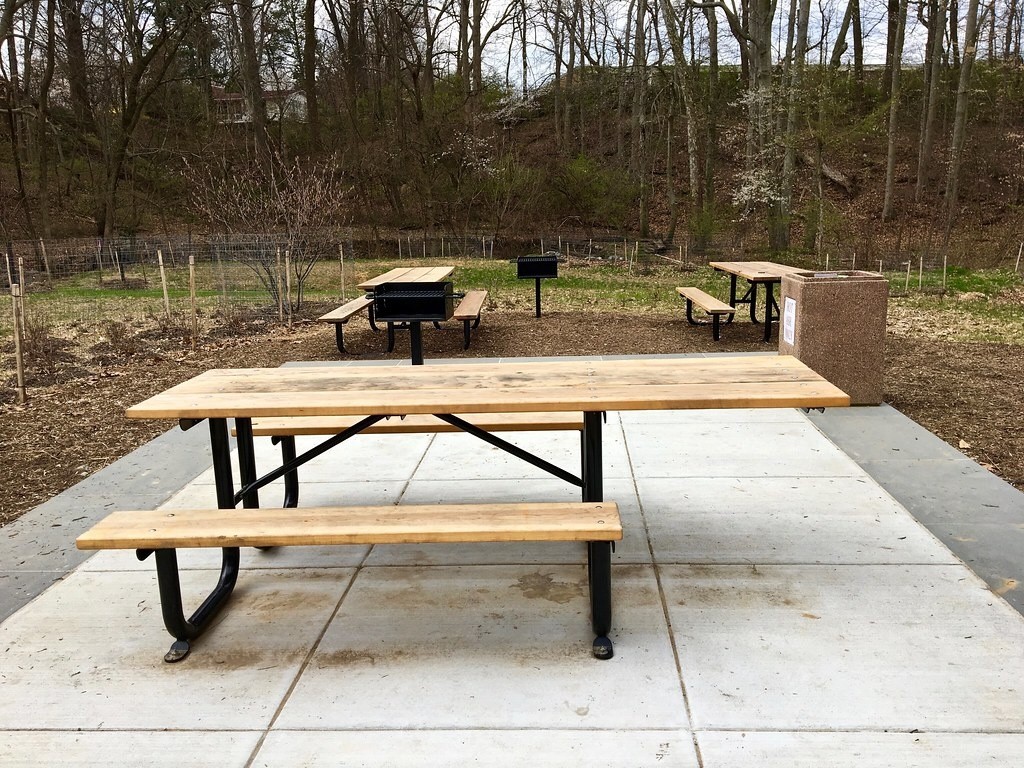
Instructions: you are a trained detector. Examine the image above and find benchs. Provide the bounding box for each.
[76,501,624,663]
[318,293,379,354]
[453,291,488,350]
[230,412,585,503]
[675,287,736,341]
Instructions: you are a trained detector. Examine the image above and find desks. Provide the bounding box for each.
[124,355,850,626]
[347,266,471,354]
[694,261,809,344]
